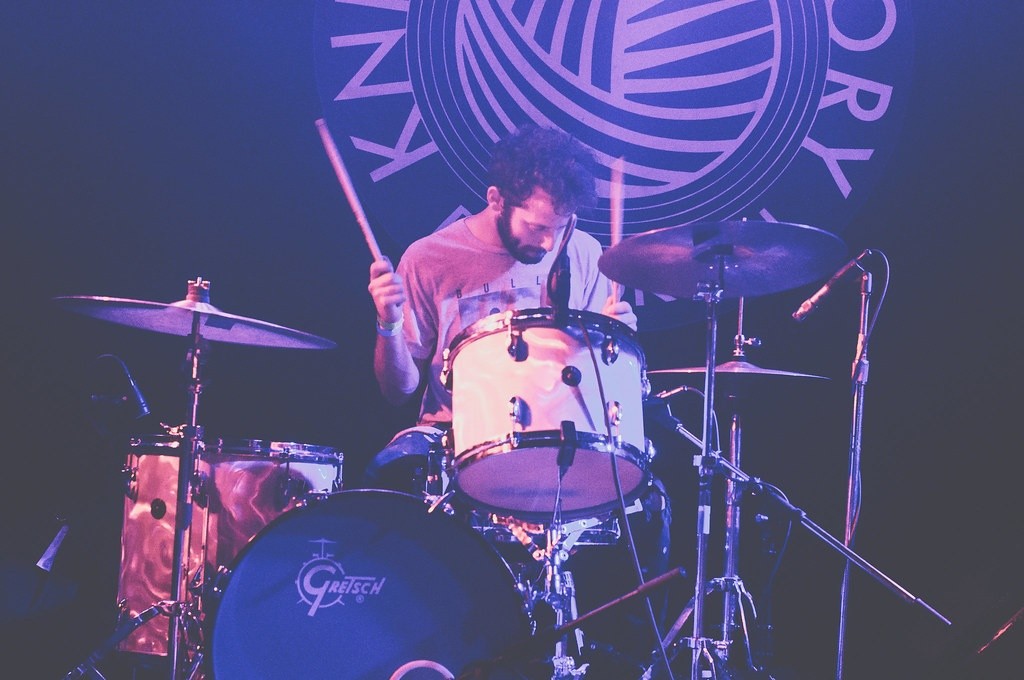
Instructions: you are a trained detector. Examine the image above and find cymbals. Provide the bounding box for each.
[596,218,852,306]
[52,292,340,350]
[649,361,830,388]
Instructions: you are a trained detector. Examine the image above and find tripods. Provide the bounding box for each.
[68,315,211,680]
[641,394,775,680]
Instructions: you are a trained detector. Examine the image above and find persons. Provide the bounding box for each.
[367,124,672,636]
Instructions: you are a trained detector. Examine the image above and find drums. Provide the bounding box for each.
[205,488,554,680]
[447,306,653,521]
[116,431,347,658]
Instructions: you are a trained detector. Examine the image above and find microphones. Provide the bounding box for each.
[120,363,151,420]
[546,243,570,309]
[792,250,873,322]
[642,386,688,413]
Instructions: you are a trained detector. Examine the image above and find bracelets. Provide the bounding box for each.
[377,312,403,329]
[375,323,402,336]
